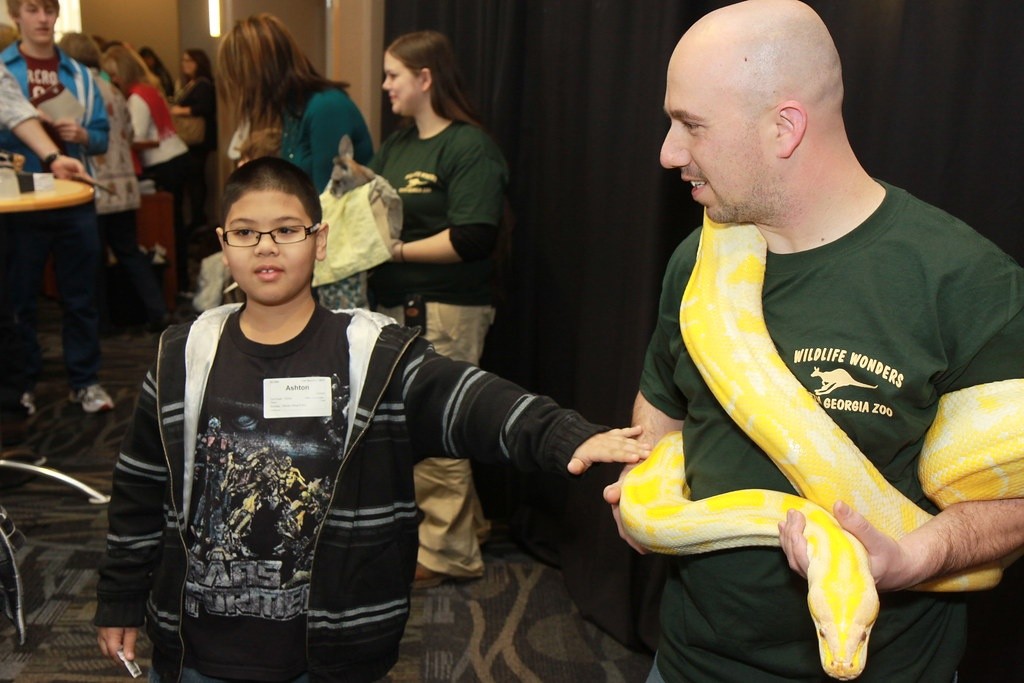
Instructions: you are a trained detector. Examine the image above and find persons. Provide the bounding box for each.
[95,156,652,683]
[216,13,372,308]
[0,64,85,180]
[61,33,217,336]
[365,30,511,581]
[0,0,115,417]
[601,1,1023,682]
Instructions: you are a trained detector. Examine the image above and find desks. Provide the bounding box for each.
[0,167,112,504]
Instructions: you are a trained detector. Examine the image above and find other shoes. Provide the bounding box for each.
[145,308,180,332]
[185,222,209,243]
[0,413,27,446]
[410,561,453,589]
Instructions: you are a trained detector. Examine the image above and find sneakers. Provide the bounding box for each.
[69,384,115,413]
[19,392,36,414]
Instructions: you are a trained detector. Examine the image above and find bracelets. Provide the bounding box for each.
[400,243,405,262]
[46,154,58,166]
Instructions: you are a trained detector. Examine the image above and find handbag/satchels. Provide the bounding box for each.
[175,115,205,145]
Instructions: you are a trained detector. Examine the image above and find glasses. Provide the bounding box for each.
[223,223,320,248]
